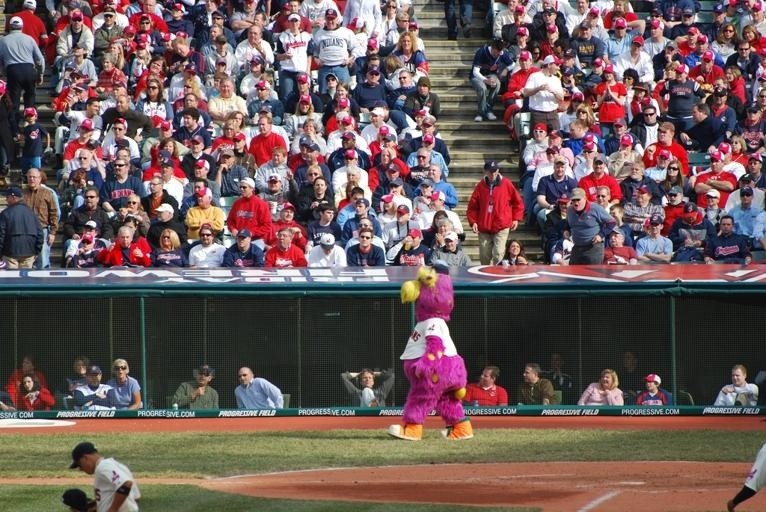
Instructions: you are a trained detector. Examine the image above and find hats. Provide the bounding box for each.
[610,227,626,238]
[643,373,661,387]
[706,100,764,199]
[636,183,684,196]
[524,118,685,162]
[85,365,102,374]
[649,214,665,225]
[570,187,587,201]
[505,4,766,100]
[683,202,698,218]
[484,159,504,171]
[0,0,458,245]
[65,440,99,469]
[198,365,214,374]
[557,192,572,204]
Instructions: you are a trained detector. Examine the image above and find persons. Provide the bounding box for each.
[233,366,284,410]
[461,364,510,407]
[70,441,144,512]
[541,351,573,389]
[0,401,16,412]
[577,369,624,407]
[17,374,55,411]
[637,373,669,406]
[516,363,559,404]
[61,355,90,393]
[340,368,395,408]
[73,365,116,409]
[106,358,142,410]
[725,441,766,511]
[0,1,766,270]
[171,361,221,409]
[618,347,646,406]
[752,371,766,386]
[712,364,760,407]
[3,353,48,404]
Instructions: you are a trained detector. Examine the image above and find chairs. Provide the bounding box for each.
[676,389,695,405]
[63,395,75,409]
[554,389,563,404]
[166,395,178,409]
[281,393,291,409]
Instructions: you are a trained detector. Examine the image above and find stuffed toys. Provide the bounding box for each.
[387,265,477,441]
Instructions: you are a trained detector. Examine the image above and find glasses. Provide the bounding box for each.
[113,365,128,370]
[576,110,588,116]
[572,98,584,104]
[596,193,610,200]
[668,166,680,171]
[643,112,656,117]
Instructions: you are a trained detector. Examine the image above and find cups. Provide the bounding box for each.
[502,259,509,266]
[270,202,277,215]
[473,399,479,406]
[630,259,637,266]
[405,241,412,250]
[172,403,178,410]
